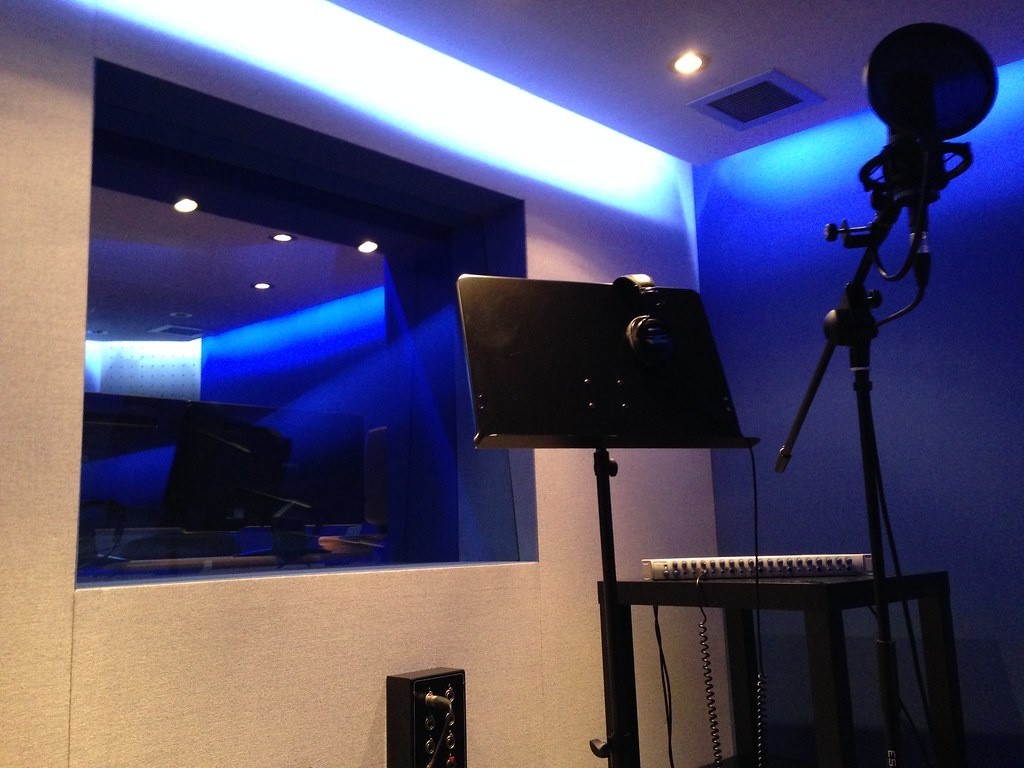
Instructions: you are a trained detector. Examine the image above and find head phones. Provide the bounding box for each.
[614,273,673,367]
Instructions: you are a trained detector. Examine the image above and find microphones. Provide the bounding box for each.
[885,76,939,290]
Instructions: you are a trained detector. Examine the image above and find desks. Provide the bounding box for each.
[597,572,968,768]
